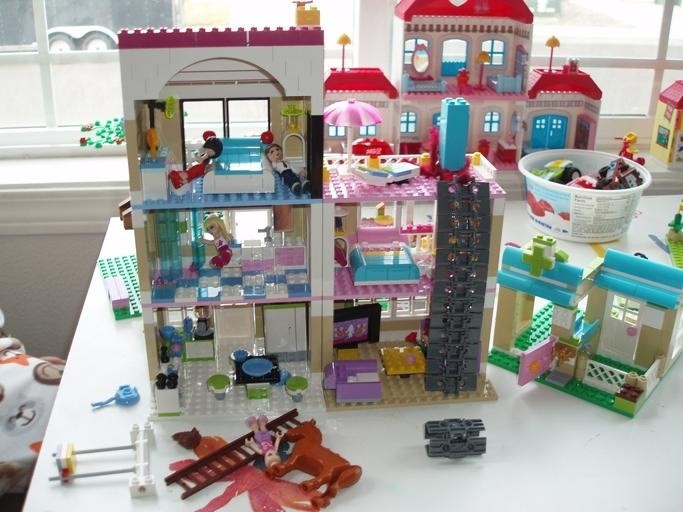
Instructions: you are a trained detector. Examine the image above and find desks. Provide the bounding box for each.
[19,196,681,512]
